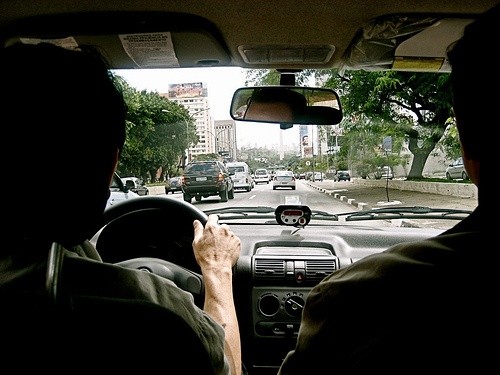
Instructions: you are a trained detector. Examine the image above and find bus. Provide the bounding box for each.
[226,162,253,192]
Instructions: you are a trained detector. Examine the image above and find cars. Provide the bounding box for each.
[112,177,149,196]
[164,177,183,194]
[445,157,470,182]
[334,171,351,183]
[375,166,393,180]
[294,171,324,182]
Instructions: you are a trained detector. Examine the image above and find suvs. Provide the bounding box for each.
[182,161,236,202]
[272,170,296,190]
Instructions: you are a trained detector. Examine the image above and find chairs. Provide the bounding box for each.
[0,72,215,375]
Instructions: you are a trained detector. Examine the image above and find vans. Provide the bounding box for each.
[254,169,270,185]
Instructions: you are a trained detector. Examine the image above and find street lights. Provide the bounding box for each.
[201,127,228,154]
[161,106,211,164]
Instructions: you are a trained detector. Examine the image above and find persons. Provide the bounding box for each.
[243,87,308,124]
[276,0,500,375]
[0,40,243,375]
